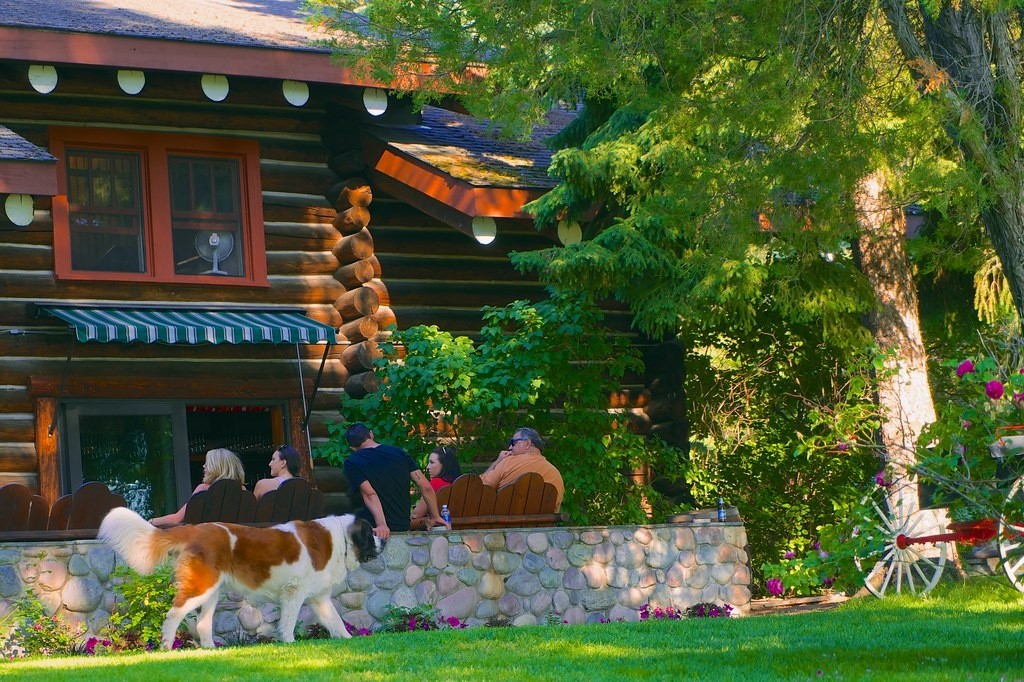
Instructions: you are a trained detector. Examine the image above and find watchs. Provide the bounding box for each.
[149,519,155,526]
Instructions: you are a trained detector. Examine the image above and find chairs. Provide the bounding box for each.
[988,423,1024,459]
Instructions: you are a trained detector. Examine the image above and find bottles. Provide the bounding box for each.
[442,503,453,532]
[717,495,727,523]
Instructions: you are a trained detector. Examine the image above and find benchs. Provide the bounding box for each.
[0,480,128,543]
[153,477,329,530]
[408,473,569,531]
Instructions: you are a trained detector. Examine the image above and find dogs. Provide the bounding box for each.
[97,505,387,653]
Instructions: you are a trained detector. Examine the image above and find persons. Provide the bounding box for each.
[411,445,463,520]
[252,444,302,501]
[147,446,246,526]
[478,428,565,516]
[342,423,451,541]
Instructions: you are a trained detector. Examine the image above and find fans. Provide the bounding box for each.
[195,230,234,275]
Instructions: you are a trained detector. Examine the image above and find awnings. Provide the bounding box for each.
[33,300,342,433]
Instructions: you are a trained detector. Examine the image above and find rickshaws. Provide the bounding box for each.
[850,430,1023,597]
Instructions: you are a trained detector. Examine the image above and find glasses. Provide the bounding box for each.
[510,437,527,446]
[442,446,447,455]
[279,443,287,459]
